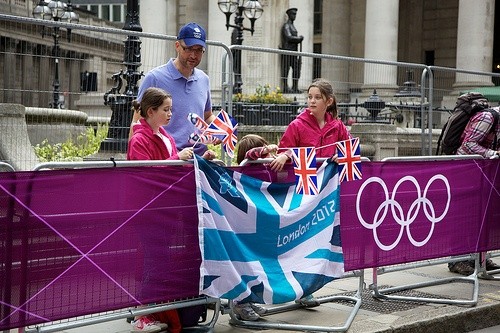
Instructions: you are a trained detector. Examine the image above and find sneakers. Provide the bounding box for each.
[250,303,267,315]
[130,319,161,333]
[145,315,168,330]
[471,259,500,270]
[230,303,260,321]
[295,295,321,307]
[448,262,474,275]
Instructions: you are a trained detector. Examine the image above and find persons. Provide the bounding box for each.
[125,23,223,325]
[128,86,219,333]
[281,8,304,94]
[448,105,500,276]
[229,134,295,320]
[275,78,353,309]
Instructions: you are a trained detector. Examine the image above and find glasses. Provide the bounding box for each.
[179,43,206,54]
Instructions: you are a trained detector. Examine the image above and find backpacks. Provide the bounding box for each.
[436,92,500,154]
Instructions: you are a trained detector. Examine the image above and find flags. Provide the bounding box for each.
[205,110,238,158]
[293,147,320,197]
[192,154,345,305]
[336,137,363,183]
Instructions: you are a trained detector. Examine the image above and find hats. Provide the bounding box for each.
[177,22,207,49]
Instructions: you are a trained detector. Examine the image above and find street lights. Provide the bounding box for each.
[32,1,79,109]
[217,0,264,94]
